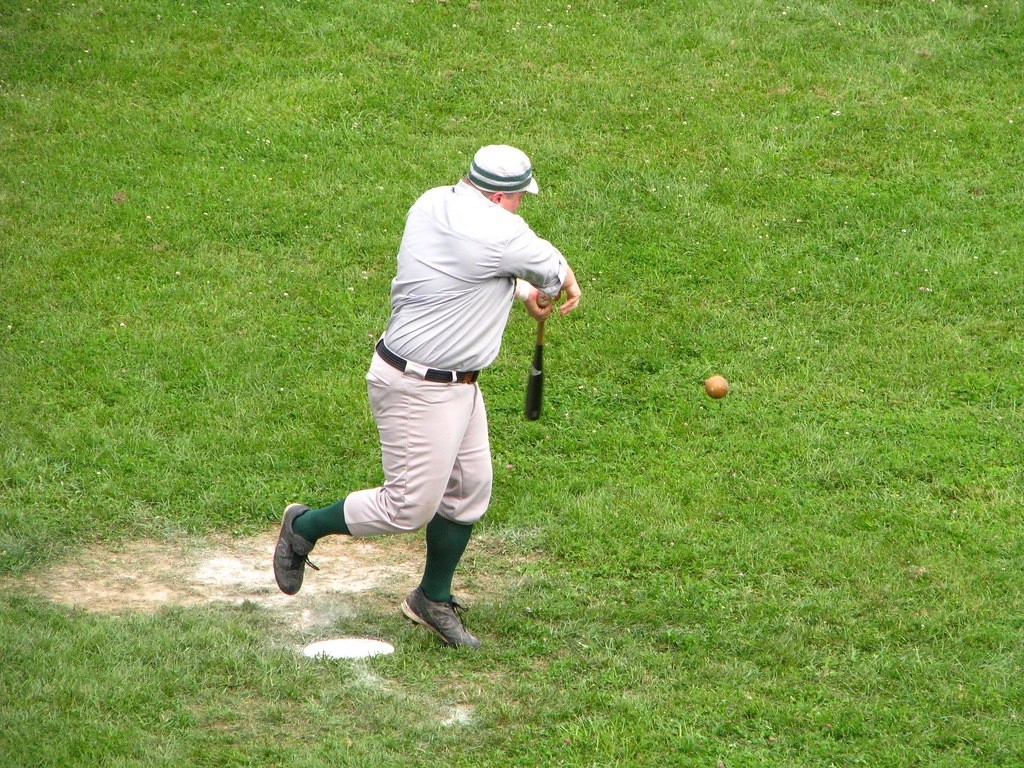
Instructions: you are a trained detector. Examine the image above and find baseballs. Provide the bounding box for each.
[705,375,730,399]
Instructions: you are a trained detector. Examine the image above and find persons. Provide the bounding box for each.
[274,145,580,650]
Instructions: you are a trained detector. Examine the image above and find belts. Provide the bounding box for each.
[375,339,479,383]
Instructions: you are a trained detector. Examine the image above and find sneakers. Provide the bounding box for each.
[273,503,320,595]
[400,586,480,650]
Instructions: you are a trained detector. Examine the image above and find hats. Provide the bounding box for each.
[466,144,539,194]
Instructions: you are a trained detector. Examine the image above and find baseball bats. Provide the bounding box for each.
[525,293,553,422]
